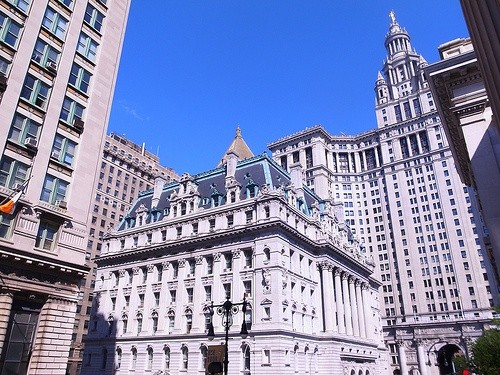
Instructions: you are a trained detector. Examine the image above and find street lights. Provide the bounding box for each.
[204,293,250,375]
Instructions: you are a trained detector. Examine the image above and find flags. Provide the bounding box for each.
[204,344,225,375]
[0,180,30,215]
